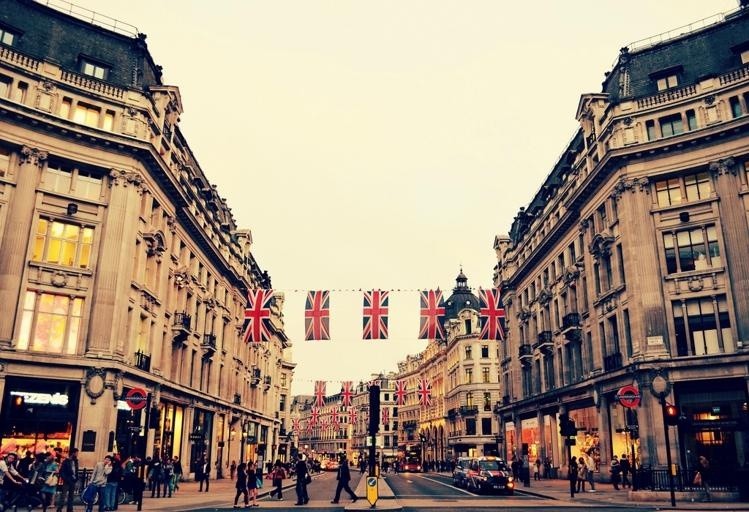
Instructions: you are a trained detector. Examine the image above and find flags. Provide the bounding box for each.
[341,381,353,406]
[292,408,390,435]
[393,380,406,405]
[479,289,505,340]
[304,290,331,341]
[362,290,389,339]
[418,289,445,339]
[242,289,273,341]
[314,381,326,407]
[418,379,431,406]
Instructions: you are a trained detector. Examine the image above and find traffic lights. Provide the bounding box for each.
[557,413,569,437]
[6,395,25,417]
[149,406,163,431]
[666,404,681,427]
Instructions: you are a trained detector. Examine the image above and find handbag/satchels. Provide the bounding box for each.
[306,475,311,483]
[44,471,58,487]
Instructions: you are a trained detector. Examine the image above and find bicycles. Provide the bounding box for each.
[79,485,127,506]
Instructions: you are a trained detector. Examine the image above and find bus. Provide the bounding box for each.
[315,449,347,472]
[395,443,422,473]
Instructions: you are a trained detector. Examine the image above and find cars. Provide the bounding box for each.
[464,455,514,495]
[451,455,474,487]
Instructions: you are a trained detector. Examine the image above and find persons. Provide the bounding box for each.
[383,458,457,473]
[692,456,713,502]
[511,453,635,498]
